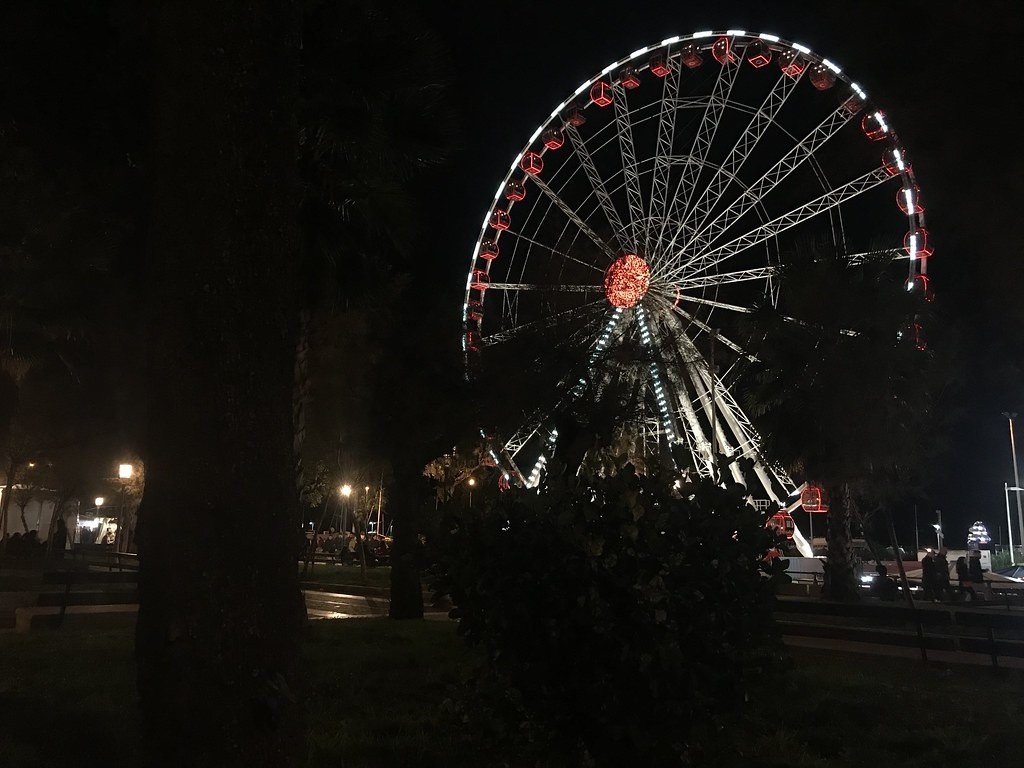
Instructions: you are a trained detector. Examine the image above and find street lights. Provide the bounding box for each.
[95,497,104,544]
[112,462,133,564]
